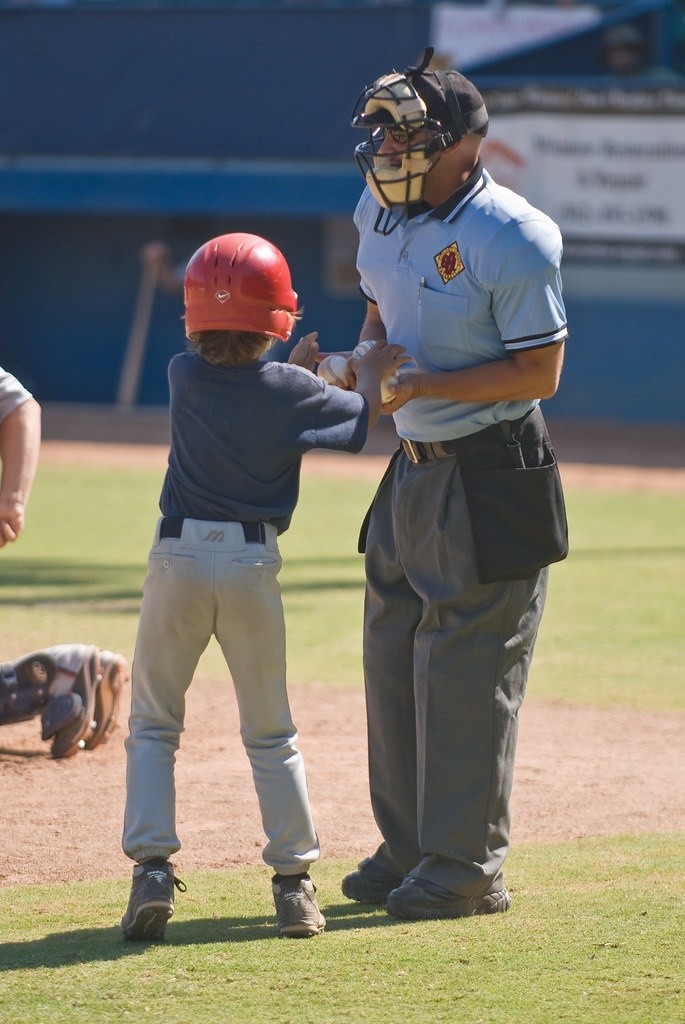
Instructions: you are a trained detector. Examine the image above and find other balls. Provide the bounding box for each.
[381,373,399,405]
[353,339,377,360]
[316,354,353,388]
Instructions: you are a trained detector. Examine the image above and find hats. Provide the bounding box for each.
[355,70,489,137]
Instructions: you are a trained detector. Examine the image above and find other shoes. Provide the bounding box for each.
[52,646,101,759]
[85,650,128,750]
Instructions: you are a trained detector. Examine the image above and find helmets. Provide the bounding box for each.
[183,232,298,342]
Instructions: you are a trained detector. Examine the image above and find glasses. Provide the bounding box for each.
[390,127,415,144]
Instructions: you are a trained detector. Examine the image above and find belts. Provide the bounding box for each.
[160,517,265,544]
[402,437,457,463]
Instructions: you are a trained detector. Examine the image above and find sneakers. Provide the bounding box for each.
[272,874,326,938]
[386,875,511,921]
[120,858,187,940]
[342,857,399,904]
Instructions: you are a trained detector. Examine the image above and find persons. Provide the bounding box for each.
[0,367,129,759]
[318,46,570,921]
[117,234,410,944]
